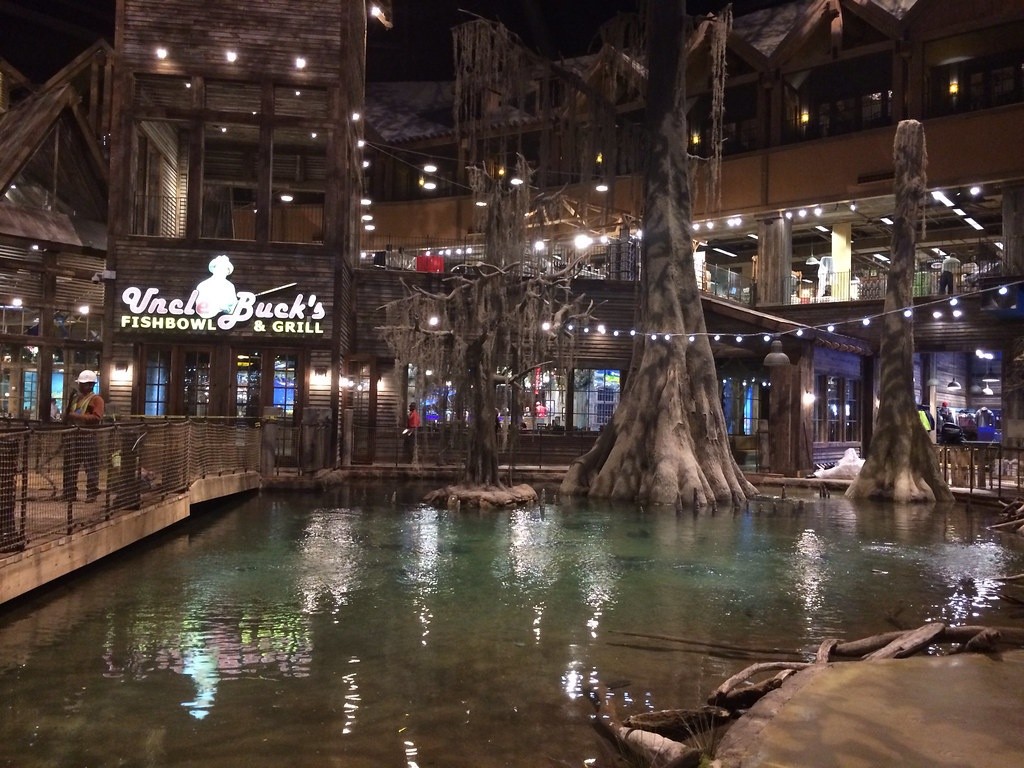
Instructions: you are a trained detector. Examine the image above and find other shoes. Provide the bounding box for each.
[54,493,76,501]
[86,494,95,504]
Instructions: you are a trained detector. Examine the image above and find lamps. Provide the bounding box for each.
[764,335,791,366]
[946,351,961,389]
[315,367,327,377]
[115,362,129,373]
[982,360,1000,382]
[927,353,941,386]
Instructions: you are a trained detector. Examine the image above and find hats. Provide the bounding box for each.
[409,402,416,406]
[536,401,540,405]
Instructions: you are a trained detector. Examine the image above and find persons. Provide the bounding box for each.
[107,439,155,509]
[383,243,410,269]
[974,407,996,429]
[494,408,501,440]
[50,399,57,418]
[245,394,259,417]
[822,285,831,297]
[54,369,104,502]
[939,402,954,423]
[404,401,420,464]
[937,251,961,295]
[535,402,548,423]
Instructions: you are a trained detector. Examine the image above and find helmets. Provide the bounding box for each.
[942,402,948,407]
[75,369,97,383]
